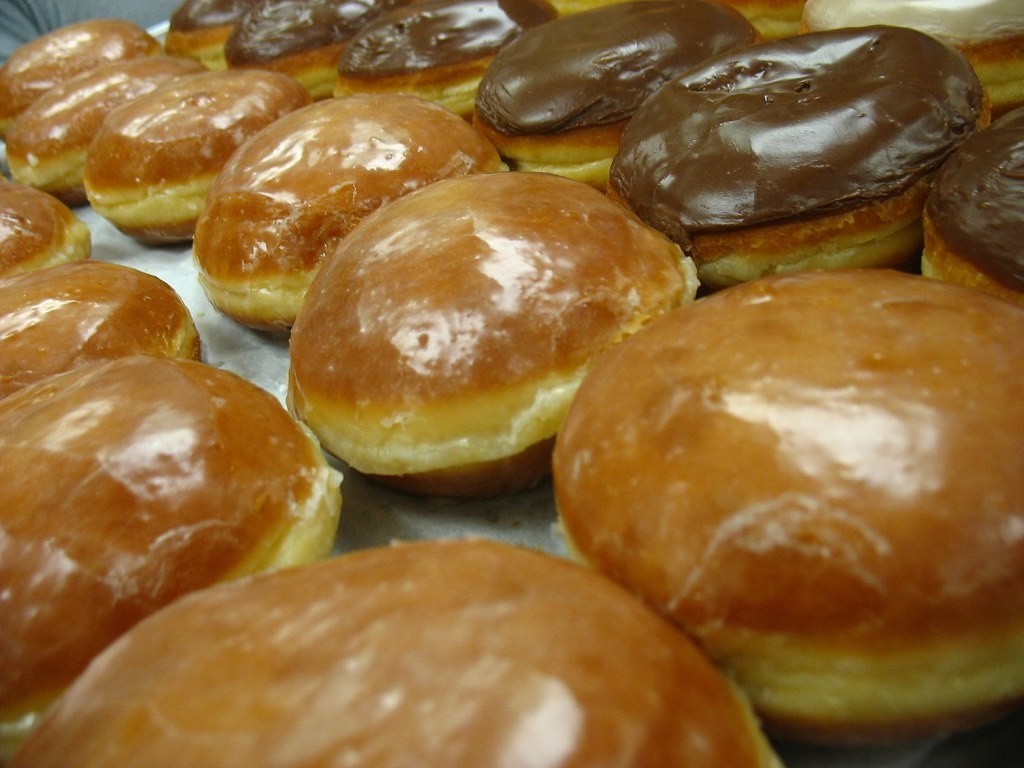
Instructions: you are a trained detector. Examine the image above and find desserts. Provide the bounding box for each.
[0,0,1024,768]
